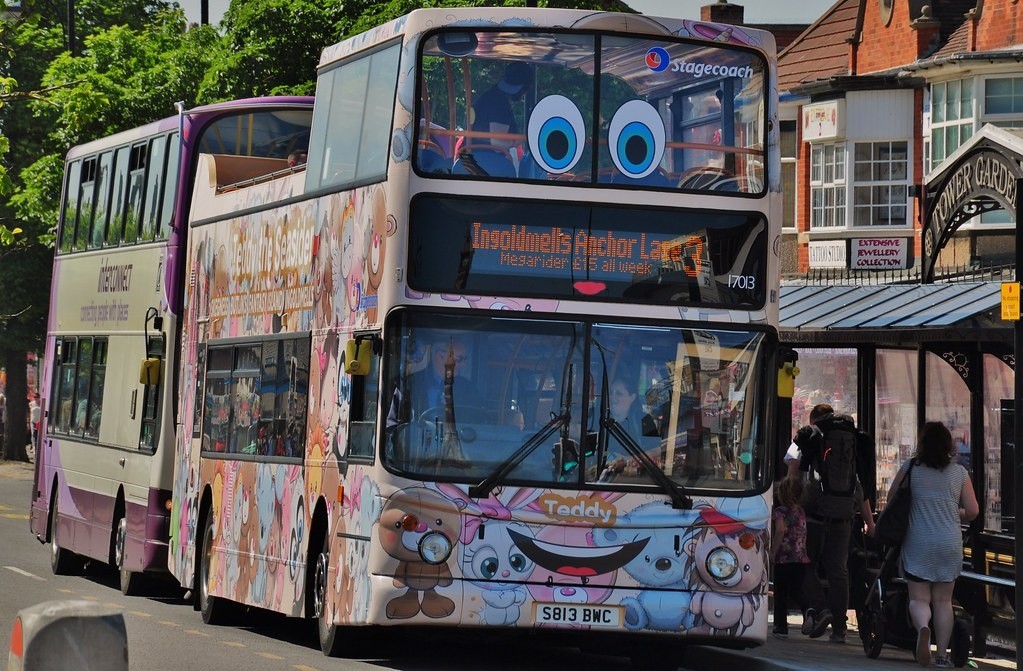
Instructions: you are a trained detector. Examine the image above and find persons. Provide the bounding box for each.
[467,61,532,153]
[885,421,979,669]
[771,478,815,637]
[58,380,102,440]
[775,401,877,643]
[385,334,484,429]
[29,393,42,453]
[587,375,661,472]
[507,408,526,431]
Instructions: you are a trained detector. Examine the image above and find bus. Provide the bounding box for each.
[29,7,800,658]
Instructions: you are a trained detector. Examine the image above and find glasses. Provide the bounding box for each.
[439,349,467,363]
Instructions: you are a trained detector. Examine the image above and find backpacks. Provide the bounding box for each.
[808,412,859,497]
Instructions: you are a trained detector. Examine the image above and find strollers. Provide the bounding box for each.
[855,524,969,668]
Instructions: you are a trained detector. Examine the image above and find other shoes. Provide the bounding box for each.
[916,625,932,667]
[772,628,788,641]
[936,654,955,667]
[830,632,846,643]
[802,621,815,635]
[809,609,833,638]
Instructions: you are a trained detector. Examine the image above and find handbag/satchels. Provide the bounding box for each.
[874,457,918,547]
